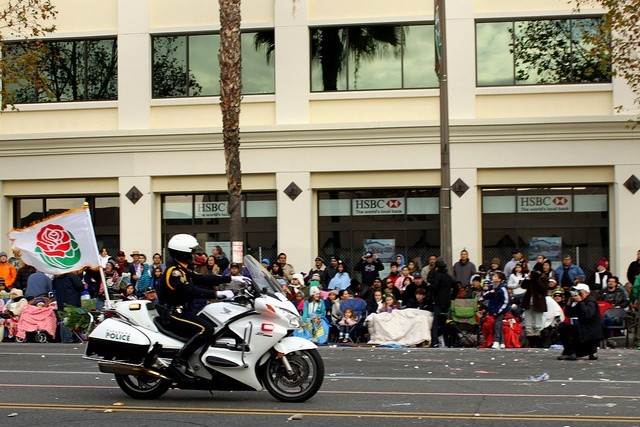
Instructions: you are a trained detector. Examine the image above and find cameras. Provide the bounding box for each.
[568,290,581,297]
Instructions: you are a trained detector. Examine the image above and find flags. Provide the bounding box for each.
[6,202,103,277]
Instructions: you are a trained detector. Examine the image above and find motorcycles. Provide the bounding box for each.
[82,254,325,402]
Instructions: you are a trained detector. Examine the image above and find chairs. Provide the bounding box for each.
[448,297,480,347]
[336,300,368,345]
[600,309,629,350]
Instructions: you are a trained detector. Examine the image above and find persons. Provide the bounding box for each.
[338,307,358,342]
[402,273,427,305]
[133,261,150,298]
[451,249,476,288]
[275,252,295,281]
[406,261,417,274]
[24,267,53,308]
[303,285,326,316]
[470,277,485,304]
[406,287,431,312]
[290,273,306,300]
[324,254,348,280]
[153,234,251,382]
[544,277,560,296]
[534,254,548,272]
[554,254,586,287]
[368,291,386,313]
[150,266,166,297]
[557,282,600,359]
[52,270,83,344]
[136,254,148,264]
[627,250,640,283]
[310,256,331,283]
[114,250,129,268]
[427,257,459,348]
[1,289,28,340]
[332,290,352,330]
[403,277,414,290]
[422,255,441,286]
[381,278,401,300]
[323,290,339,313]
[600,276,630,335]
[291,292,306,311]
[194,252,210,274]
[632,273,639,312]
[225,262,243,277]
[128,251,146,277]
[98,247,111,261]
[540,260,554,281]
[0,252,18,293]
[484,273,508,348]
[394,267,415,292]
[507,262,532,301]
[485,258,504,284]
[305,273,325,289]
[121,282,137,302]
[385,262,402,285]
[200,256,222,275]
[379,294,400,314]
[395,253,407,272]
[1,277,11,341]
[147,252,168,273]
[327,263,353,289]
[354,251,384,284]
[504,251,532,284]
[259,257,271,271]
[98,261,121,297]
[211,246,229,265]
[269,263,288,290]
[550,290,572,325]
[587,258,611,290]
[362,279,388,313]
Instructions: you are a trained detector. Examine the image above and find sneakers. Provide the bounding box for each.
[171,356,196,383]
[590,353,598,360]
[491,342,499,349]
[557,353,576,360]
[500,344,505,349]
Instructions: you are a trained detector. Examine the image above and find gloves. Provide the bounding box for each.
[231,276,252,286]
[216,290,234,300]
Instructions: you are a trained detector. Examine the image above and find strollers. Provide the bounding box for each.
[16,293,54,343]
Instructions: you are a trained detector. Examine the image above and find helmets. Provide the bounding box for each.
[167,233,203,252]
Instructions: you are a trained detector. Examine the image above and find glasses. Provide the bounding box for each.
[315,260,321,261]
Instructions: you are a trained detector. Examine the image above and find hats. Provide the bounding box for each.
[309,286,320,297]
[230,262,239,268]
[364,252,373,258]
[291,274,304,285]
[130,251,140,256]
[261,259,270,264]
[491,259,501,265]
[570,283,590,293]
[533,262,543,273]
[551,287,565,302]
[329,290,339,295]
[316,256,325,263]
[482,279,493,291]
[596,256,608,268]
